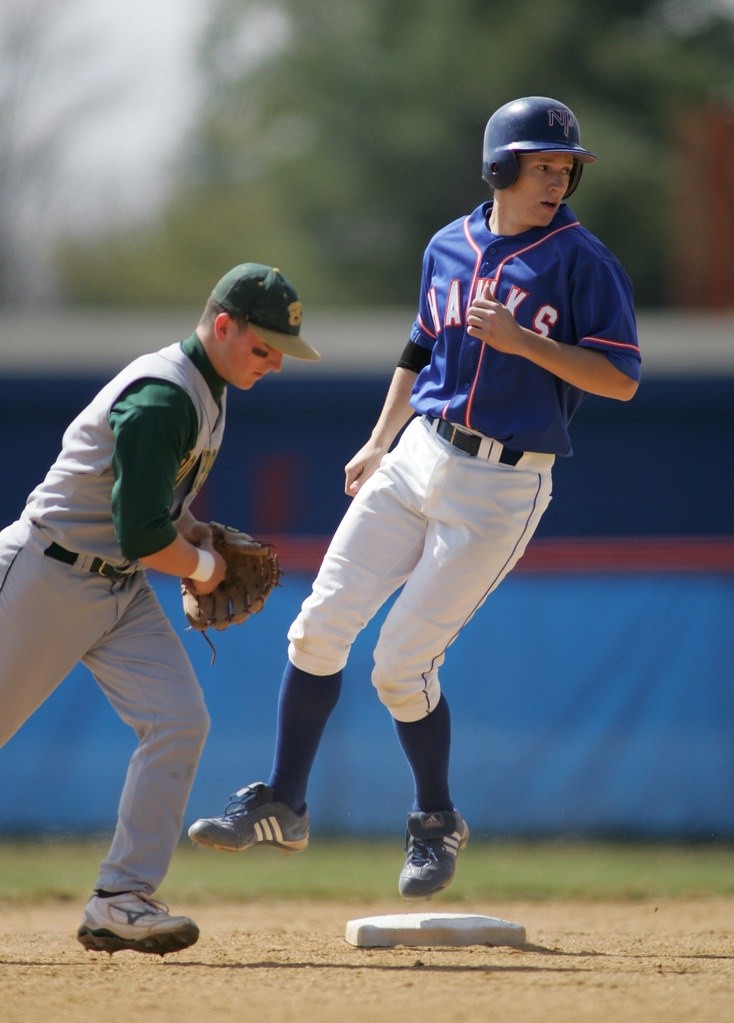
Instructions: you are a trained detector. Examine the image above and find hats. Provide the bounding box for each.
[210,262,321,361]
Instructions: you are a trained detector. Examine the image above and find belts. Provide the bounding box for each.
[43,540,131,582]
[425,413,523,468]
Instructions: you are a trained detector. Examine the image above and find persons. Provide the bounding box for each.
[189,95,641,898]
[0,263,320,957]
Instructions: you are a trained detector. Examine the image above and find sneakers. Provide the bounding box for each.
[397,801,470,901]
[77,891,200,957]
[187,781,310,854]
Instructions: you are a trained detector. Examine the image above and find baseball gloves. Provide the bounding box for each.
[184,520,280,633]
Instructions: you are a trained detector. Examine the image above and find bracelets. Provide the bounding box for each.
[186,544,214,583]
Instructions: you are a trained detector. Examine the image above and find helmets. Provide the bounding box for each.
[481,96,599,200]
